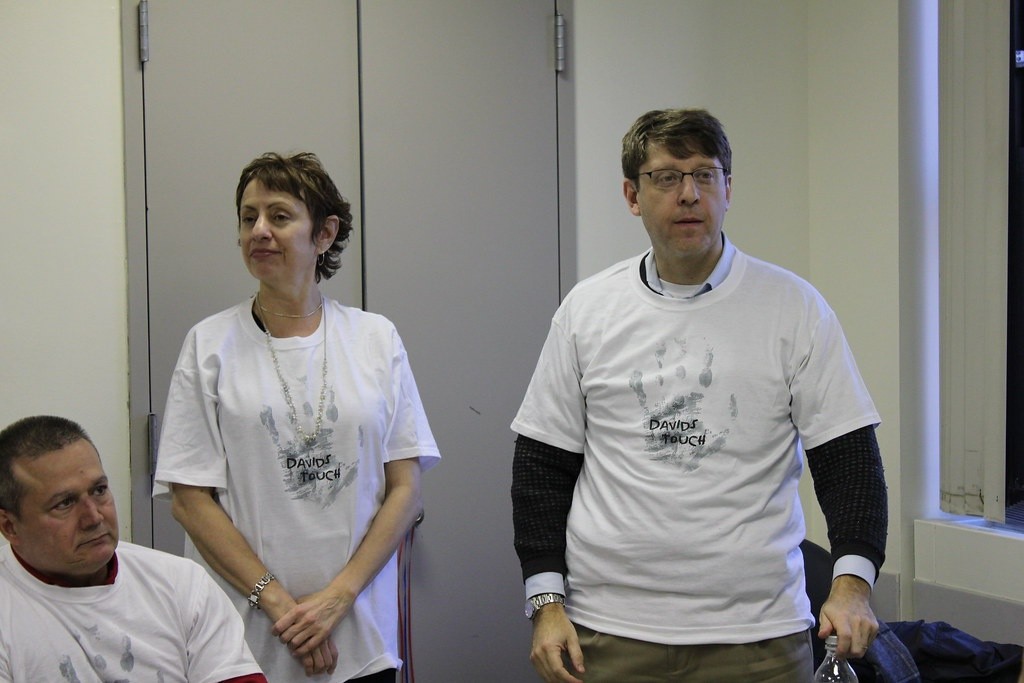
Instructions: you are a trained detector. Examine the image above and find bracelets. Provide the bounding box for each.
[247,572,277,609]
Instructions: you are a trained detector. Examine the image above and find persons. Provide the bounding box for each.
[0,415,267,682]
[510,110,889,683]
[150,150,442,683]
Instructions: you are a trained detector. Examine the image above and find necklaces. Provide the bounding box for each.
[256,290,328,443]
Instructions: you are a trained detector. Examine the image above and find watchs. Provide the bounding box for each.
[525,593,566,620]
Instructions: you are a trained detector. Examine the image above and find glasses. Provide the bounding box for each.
[632,167,728,190]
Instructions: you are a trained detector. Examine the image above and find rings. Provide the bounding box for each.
[862,647,868,649]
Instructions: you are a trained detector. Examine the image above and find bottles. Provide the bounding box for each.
[815,636,859,683]
